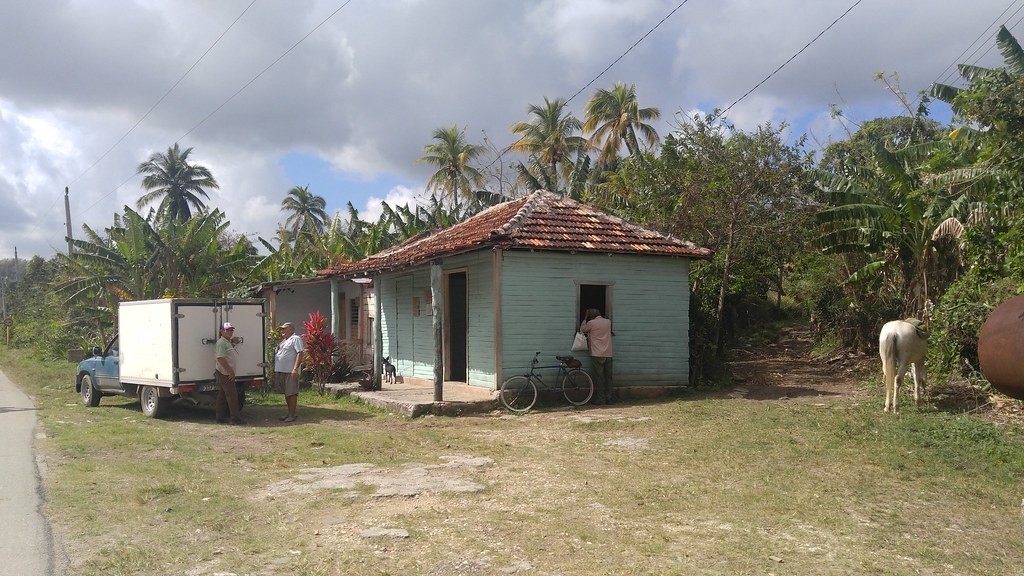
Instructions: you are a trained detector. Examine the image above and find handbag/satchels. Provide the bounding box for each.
[571,328,589,351]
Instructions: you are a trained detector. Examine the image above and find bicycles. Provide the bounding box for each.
[499,351,596,415]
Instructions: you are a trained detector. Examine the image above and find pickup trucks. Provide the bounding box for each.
[74,298,267,417]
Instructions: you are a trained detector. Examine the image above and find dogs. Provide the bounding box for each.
[381,356,396,384]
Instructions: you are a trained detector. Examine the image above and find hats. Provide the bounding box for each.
[279,323,295,330]
[220,323,235,330]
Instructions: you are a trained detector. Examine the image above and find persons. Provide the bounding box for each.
[215,322,248,424]
[581,304,614,405]
[275,322,304,422]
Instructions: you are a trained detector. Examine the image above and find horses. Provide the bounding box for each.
[879,318,928,415]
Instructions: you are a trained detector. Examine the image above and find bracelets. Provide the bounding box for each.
[294,369,296,370]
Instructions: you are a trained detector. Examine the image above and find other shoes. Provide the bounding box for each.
[279,415,289,421]
[591,400,603,405]
[215,418,230,424]
[606,398,614,405]
[230,417,246,426]
[284,416,297,422]
